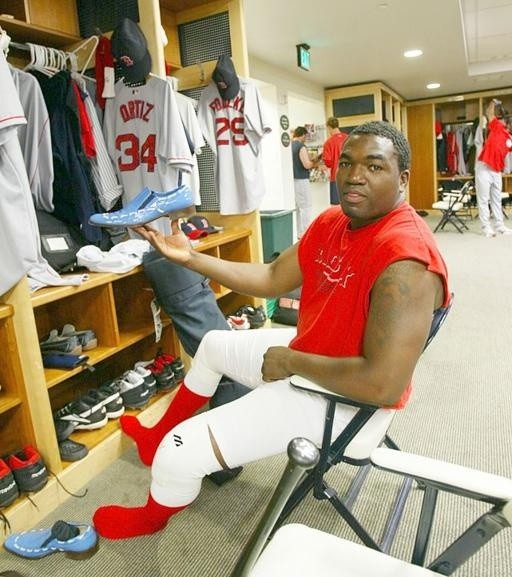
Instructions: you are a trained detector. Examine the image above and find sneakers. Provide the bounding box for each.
[52,348,185,462]
[494,226,512,235]
[0,446,49,500]
[224,304,266,329]
[481,225,496,238]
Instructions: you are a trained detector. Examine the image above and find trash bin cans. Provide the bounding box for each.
[260,209,299,264]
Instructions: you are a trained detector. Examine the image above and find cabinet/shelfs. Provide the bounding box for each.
[0,223,255,551]
[432,87,512,219]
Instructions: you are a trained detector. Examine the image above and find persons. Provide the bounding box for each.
[91,119,449,540]
[474,98,512,238]
[291,126,320,240]
[320,116,349,206]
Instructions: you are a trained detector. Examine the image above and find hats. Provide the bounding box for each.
[109,16,151,84]
[181,215,219,239]
[211,53,240,100]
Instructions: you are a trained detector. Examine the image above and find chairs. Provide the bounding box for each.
[430,180,510,234]
[249,448,511,577]
[268,292,454,568]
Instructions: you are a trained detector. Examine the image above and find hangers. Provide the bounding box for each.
[24,35,99,83]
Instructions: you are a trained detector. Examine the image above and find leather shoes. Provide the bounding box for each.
[4,523,100,560]
[39,325,98,355]
[87,184,196,229]
